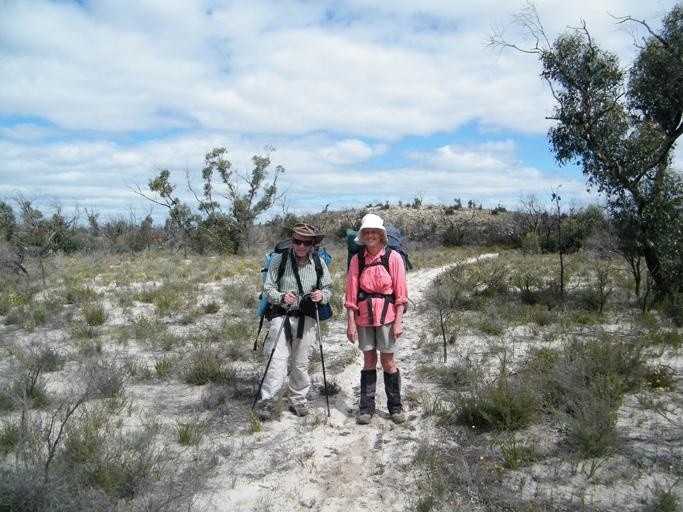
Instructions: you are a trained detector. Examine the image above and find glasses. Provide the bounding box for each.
[292,237,313,246]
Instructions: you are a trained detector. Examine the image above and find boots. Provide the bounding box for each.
[357,370,376,424]
[385,369,405,423]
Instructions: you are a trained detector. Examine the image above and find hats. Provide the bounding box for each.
[284,223,325,245]
[353,214,388,245]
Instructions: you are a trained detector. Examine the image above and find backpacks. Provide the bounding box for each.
[256,239,332,315]
[345,226,412,305]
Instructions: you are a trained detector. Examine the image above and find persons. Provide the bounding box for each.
[341,212,410,425]
[253,220,335,422]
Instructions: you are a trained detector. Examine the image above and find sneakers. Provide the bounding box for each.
[289,404,308,417]
[260,400,273,419]
